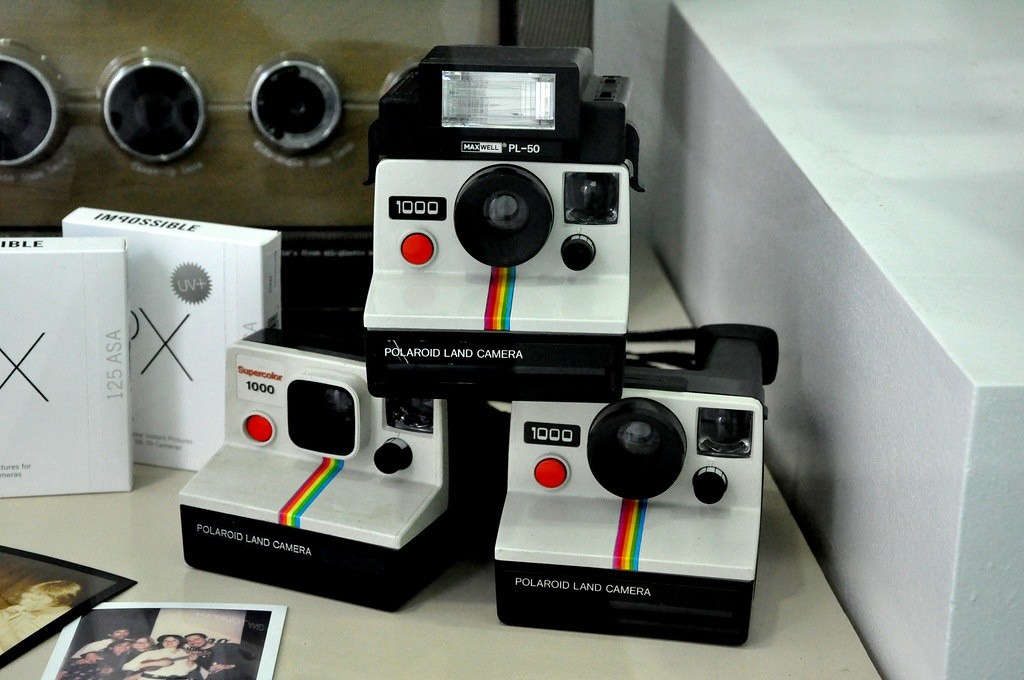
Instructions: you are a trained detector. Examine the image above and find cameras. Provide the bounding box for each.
[176,46,779,646]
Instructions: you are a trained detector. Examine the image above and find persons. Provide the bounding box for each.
[0,579,81,656]
[56,626,259,680]
